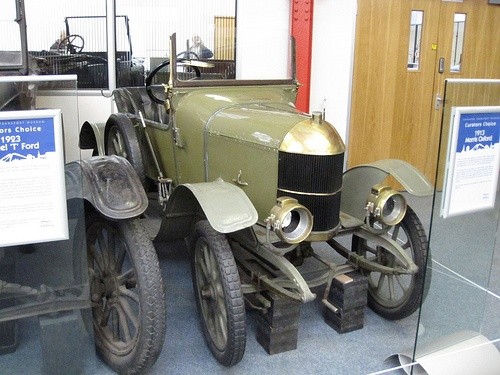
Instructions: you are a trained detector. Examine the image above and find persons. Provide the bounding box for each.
[50,33,77,55]
[184,36,213,60]
